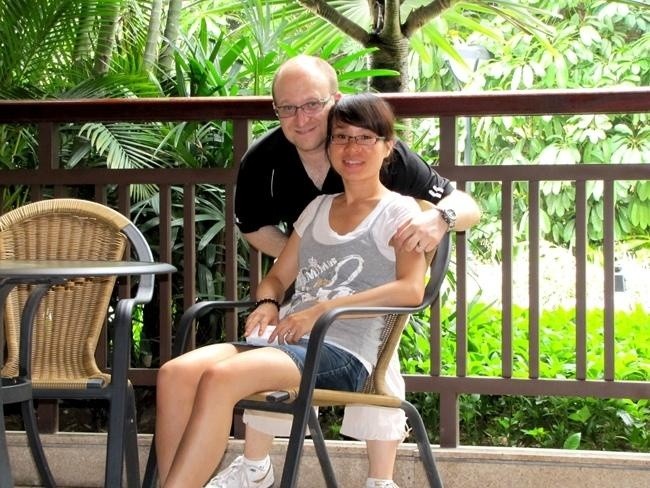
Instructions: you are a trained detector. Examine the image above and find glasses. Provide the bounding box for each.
[273,96,334,119]
[329,132,388,146]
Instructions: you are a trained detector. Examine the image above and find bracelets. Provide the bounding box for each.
[254,298,280,313]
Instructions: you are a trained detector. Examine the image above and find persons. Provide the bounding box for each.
[201,52,480,488]
[155,92,426,488]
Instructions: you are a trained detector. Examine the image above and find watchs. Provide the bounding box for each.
[434,204,457,233]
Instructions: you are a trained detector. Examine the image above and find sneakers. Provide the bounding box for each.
[206,454,274,488]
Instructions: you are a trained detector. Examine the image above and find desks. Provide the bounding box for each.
[1,263,178,487]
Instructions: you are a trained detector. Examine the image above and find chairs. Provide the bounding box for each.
[0,197,156,488]
[174,196,453,487]
